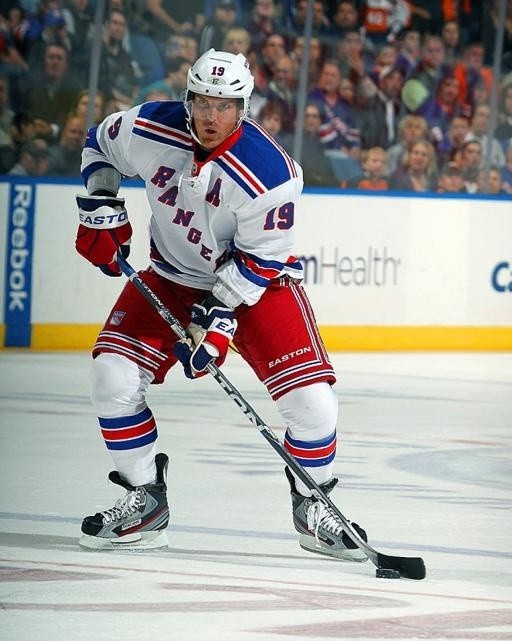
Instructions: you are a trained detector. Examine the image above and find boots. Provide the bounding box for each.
[81,454,170,538]
[284,465,367,549]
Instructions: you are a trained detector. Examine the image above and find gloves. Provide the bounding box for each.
[74,194,133,278]
[174,302,239,381]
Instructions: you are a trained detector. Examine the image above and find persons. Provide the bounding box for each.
[71,47,369,550]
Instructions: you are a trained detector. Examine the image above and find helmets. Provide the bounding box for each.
[184,47,255,116]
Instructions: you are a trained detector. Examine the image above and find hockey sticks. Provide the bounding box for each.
[113,251,426,582]
